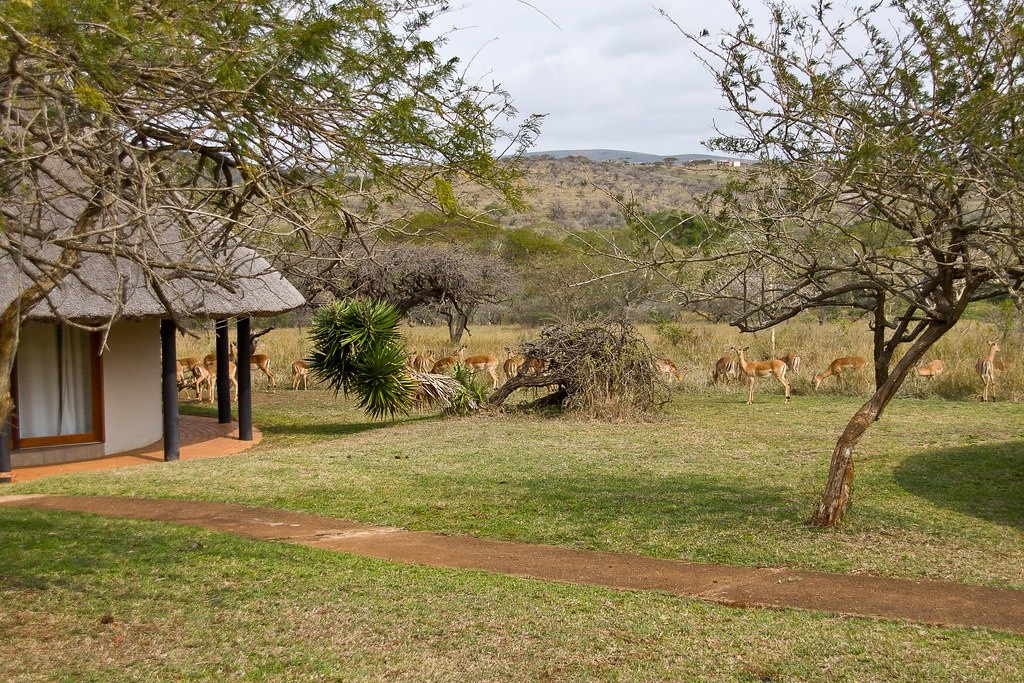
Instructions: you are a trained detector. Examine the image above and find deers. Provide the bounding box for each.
[713,345,801,405]
[403,346,559,393]
[912,356,945,393]
[811,357,870,396]
[641,351,682,385]
[975,339,1001,403]
[174,340,317,405]
